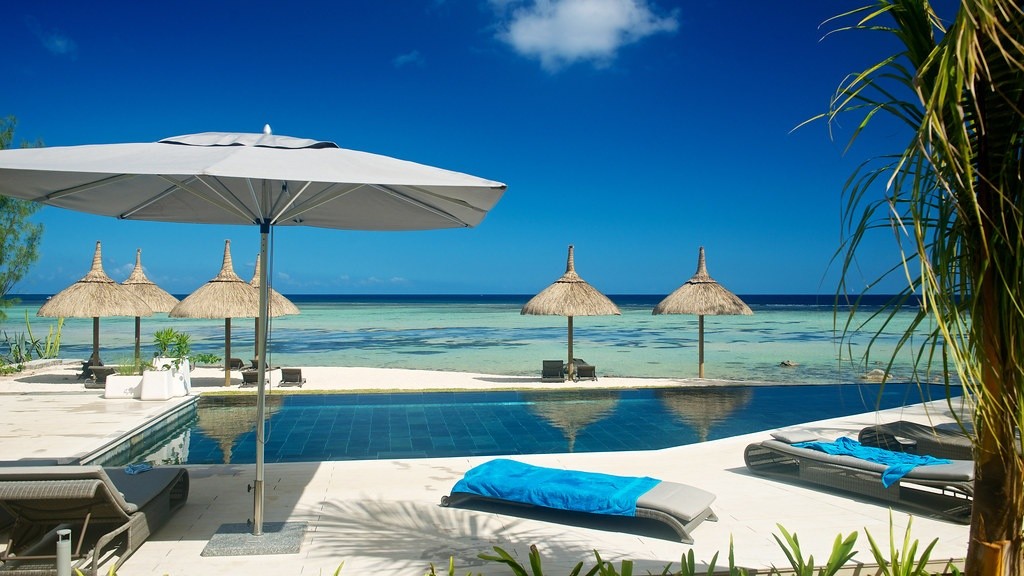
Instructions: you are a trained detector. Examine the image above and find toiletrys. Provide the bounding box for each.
[125,460,155,474]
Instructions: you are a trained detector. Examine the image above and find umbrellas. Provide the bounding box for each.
[122,249,180,366]
[195,396,280,465]
[528,394,617,452]
[35,242,151,378]
[168,239,260,387]
[0,124,507,534]
[249,253,300,359]
[521,245,619,381]
[653,248,754,377]
[662,390,750,443]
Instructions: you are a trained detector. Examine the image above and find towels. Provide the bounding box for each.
[790,437,953,489]
[450,459,661,517]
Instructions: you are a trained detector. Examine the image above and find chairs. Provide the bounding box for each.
[248,359,281,372]
[576,365,598,381]
[278,368,307,388]
[437,457,720,545]
[76,361,103,380]
[84,366,115,389]
[744,419,1022,526]
[238,371,269,388]
[541,359,566,383]
[0,465,190,576]
[220,357,252,371]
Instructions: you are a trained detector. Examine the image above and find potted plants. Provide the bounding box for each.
[107,327,222,400]
